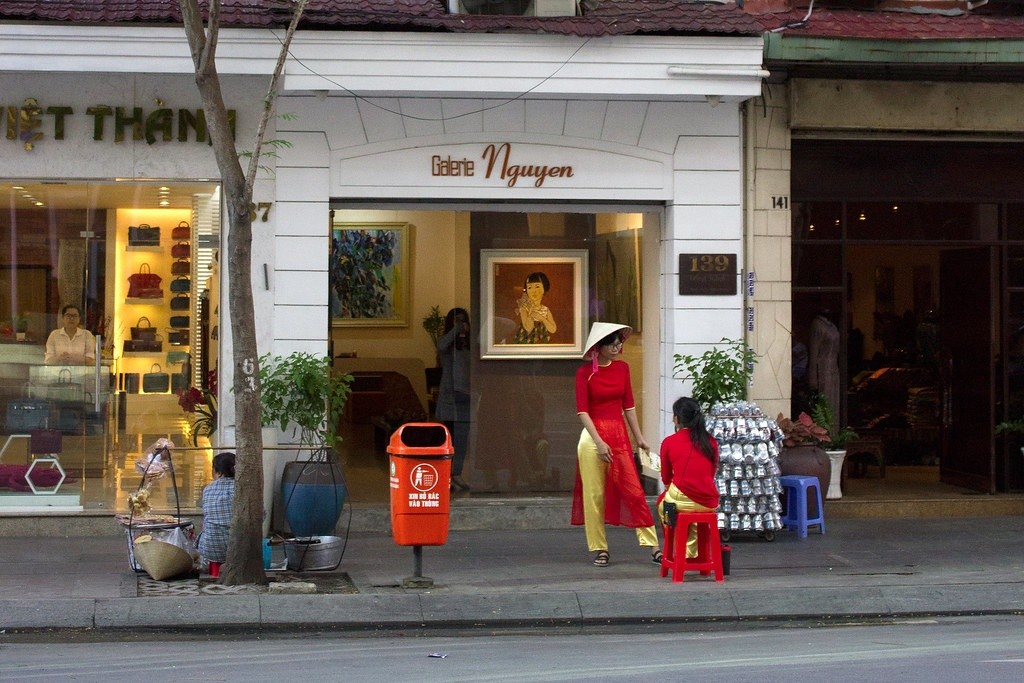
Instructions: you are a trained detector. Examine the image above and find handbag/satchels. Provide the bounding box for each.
[164,527,193,559]
[130,317,157,340]
[143,363,169,393]
[124,372,140,394]
[128,263,163,297]
[123,339,148,352]
[168,221,191,393]
[6,368,97,454]
[128,224,160,246]
[663,501,676,525]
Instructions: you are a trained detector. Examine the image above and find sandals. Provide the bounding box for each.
[594,551,609,566]
[651,550,663,564]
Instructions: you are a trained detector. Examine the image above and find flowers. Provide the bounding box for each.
[382,407,425,425]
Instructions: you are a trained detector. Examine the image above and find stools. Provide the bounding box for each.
[660,511,725,583]
[776,475,826,539]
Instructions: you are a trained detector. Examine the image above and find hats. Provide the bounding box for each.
[582,322,633,361]
[134,541,193,580]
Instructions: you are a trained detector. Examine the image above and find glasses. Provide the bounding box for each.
[607,343,623,350]
[65,314,80,319]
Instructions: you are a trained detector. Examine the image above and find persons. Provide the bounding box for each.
[571,322,665,566]
[808,311,840,433]
[476,361,549,495]
[192,452,267,578]
[656,396,720,559]
[433,308,471,493]
[44,304,97,366]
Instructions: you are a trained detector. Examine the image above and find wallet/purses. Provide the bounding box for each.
[139,288,163,299]
[149,341,163,352]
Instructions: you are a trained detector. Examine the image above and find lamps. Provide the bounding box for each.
[873,310,896,356]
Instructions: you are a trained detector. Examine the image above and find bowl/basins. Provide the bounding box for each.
[284,535,342,571]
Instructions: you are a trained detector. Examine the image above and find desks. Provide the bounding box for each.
[369,415,392,463]
[841,437,886,480]
[425,367,455,462]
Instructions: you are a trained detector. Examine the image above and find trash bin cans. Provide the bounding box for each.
[386,422,455,546]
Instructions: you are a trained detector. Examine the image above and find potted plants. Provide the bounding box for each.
[15,320,27,342]
[231,352,356,542]
[771,408,832,528]
[808,391,860,501]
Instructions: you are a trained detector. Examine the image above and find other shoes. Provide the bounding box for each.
[452,478,469,490]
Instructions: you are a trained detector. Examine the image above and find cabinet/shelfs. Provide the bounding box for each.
[105,206,210,437]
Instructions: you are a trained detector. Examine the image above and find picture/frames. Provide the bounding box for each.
[331,222,410,327]
[479,248,589,360]
[874,264,894,311]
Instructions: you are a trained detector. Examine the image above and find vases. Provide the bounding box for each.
[434,350,442,368]
